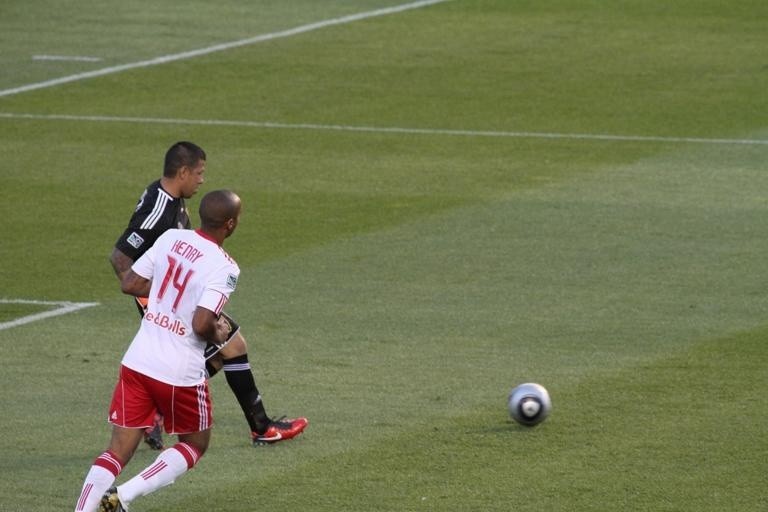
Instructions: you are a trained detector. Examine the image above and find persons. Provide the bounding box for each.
[72,189,245,511]
[107,137,310,451]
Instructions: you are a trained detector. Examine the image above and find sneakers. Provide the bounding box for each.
[143,415,166,450]
[100,486,126,512]
[251,417,308,446]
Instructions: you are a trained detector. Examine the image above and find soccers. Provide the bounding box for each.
[508,380,553,428]
